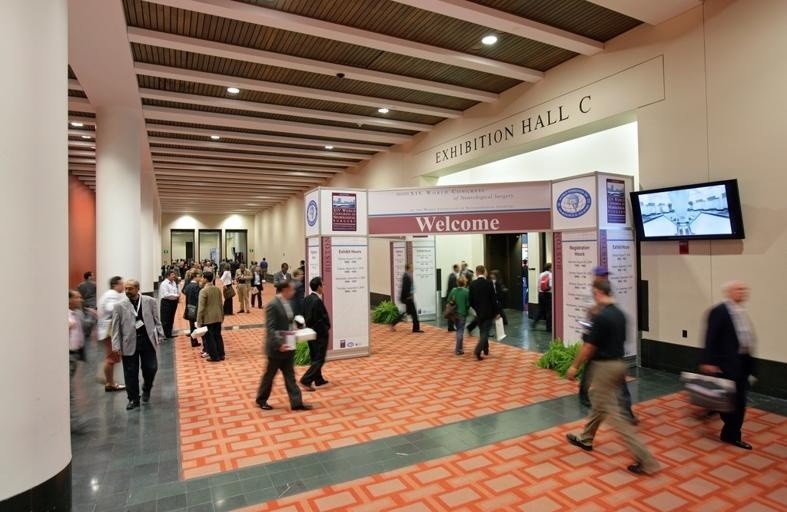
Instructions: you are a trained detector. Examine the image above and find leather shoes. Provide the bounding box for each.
[447,326,494,360]
[142,386,150,402]
[628,463,645,473]
[105,383,126,391]
[579,392,591,407]
[256,380,329,410]
[126,399,140,409]
[413,328,424,333]
[566,434,592,450]
[720,435,752,449]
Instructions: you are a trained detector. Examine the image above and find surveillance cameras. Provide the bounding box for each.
[337,73,344,78]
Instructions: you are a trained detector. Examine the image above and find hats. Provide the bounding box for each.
[594,266,610,275]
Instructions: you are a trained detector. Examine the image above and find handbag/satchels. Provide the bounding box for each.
[183,304,198,322]
[444,300,458,322]
[223,286,236,299]
[495,317,507,342]
[680,371,736,413]
[541,274,552,292]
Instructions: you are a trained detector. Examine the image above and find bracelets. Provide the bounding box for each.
[569,365,578,371]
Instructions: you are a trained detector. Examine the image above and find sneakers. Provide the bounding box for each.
[186,332,226,361]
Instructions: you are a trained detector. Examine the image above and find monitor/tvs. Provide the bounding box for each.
[629,178,744,242]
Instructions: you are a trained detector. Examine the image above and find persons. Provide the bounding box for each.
[253,278,314,411]
[522,258,529,301]
[75,271,96,338]
[697,279,758,451]
[300,275,332,391]
[94,275,129,391]
[389,263,424,333]
[530,262,552,332]
[69,289,99,437]
[562,276,660,476]
[157,257,308,363]
[109,278,164,411]
[442,259,511,361]
[578,272,639,424]
